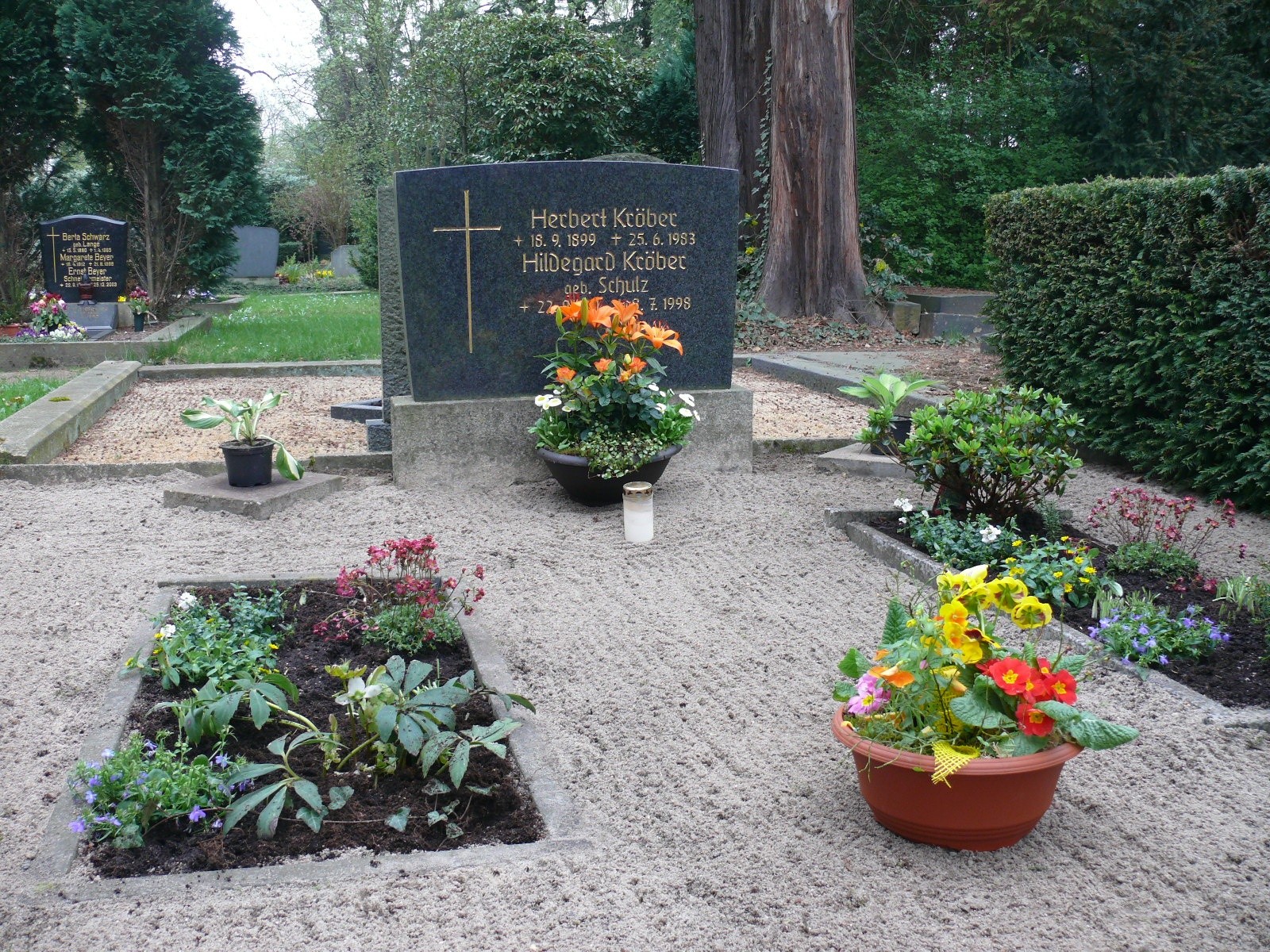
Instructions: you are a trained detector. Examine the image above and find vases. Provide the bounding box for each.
[831,699,1087,852]
[47,322,58,334]
[538,443,681,505]
[133,311,144,332]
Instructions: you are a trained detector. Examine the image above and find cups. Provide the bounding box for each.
[621,481,656,543]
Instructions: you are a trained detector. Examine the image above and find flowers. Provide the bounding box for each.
[833,560,1141,782]
[526,292,704,481]
[17,289,89,342]
[128,285,157,327]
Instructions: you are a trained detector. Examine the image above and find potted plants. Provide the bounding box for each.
[0,263,37,336]
[179,388,305,489]
